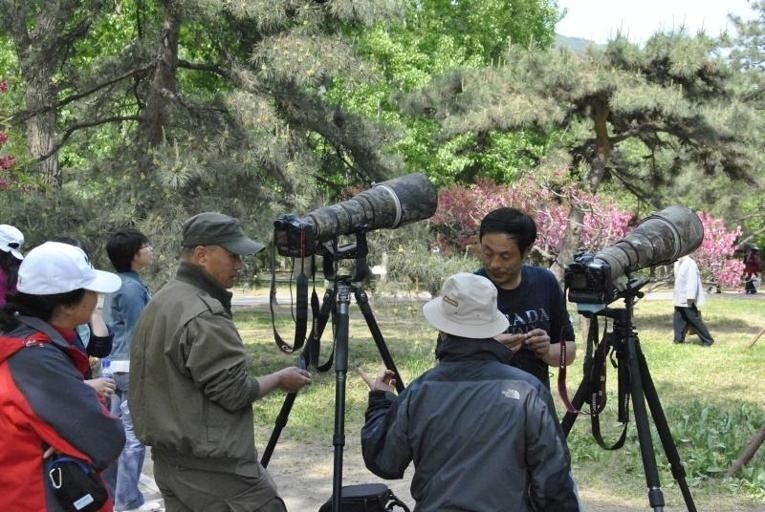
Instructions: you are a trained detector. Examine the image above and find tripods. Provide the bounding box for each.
[558,304,697,512]
[259,260,405,512]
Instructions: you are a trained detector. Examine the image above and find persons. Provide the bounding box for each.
[434,207,575,394]
[1,223,25,305]
[1,240,125,511]
[355,273,583,511]
[96,229,160,511]
[129,211,313,511]
[70,293,111,381]
[673,250,714,347]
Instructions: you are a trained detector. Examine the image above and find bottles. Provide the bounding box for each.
[96,360,112,410]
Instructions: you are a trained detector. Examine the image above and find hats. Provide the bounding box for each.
[423,272,510,339]
[0,224,25,260]
[17,241,121,295]
[181,212,266,256]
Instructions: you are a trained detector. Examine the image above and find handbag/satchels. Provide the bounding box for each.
[46,457,108,512]
